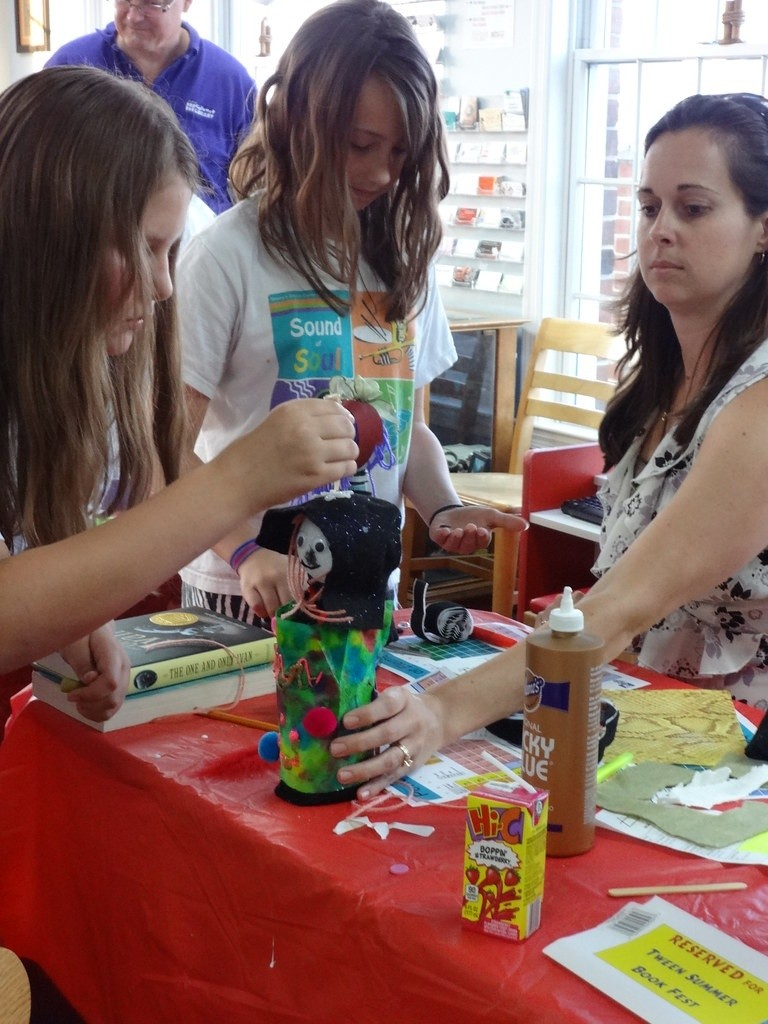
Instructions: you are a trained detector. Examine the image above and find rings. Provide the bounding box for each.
[394,741,413,768]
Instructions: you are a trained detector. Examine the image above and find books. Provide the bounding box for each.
[29,605,278,734]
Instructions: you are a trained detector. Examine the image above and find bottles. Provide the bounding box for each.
[523,584,605,855]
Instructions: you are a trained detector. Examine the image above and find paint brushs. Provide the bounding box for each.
[192,706,280,732]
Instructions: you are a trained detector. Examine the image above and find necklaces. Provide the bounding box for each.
[660,411,668,422]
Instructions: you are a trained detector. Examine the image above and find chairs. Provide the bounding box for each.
[391,318,644,626]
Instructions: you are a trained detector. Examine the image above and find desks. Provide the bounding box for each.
[518,442,607,623]
[420,307,529,470]
[421,308,533,471]
[1,602,768,1024]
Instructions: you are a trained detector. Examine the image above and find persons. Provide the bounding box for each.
[332,93,768,799]
[0,65,360,723]
[44,1,258,215]
[176,0,530,638]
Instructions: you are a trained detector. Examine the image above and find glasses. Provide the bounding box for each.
[707,92,768,120]
[114,0,175,17]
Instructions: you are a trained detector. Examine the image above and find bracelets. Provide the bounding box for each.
[428,504,464,527]
[228,539,259,576]
